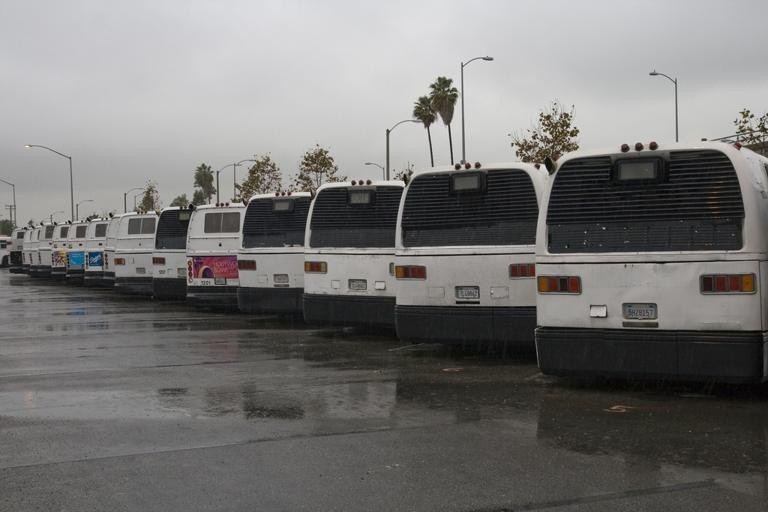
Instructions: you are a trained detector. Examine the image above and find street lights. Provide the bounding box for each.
[457,55,493,165]
[381,119,423,181]
[124,187,141,212]
[77,198,95,222]
[134,190,148,212]
[214,163,241,205]
[24,142,75,224]
[649,68,680,146]
[231,159,257,205]
[364,160,386,183]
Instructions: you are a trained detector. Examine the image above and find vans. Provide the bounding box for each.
[529,141,766,405]
[85,211,114,275]
[299,177,411,333]
[235,189,314,317]
[379,156,553,357]
[152,205,198,300]
[186,202,241,290]
[112,206,154,284]
[14,223,87,276]
